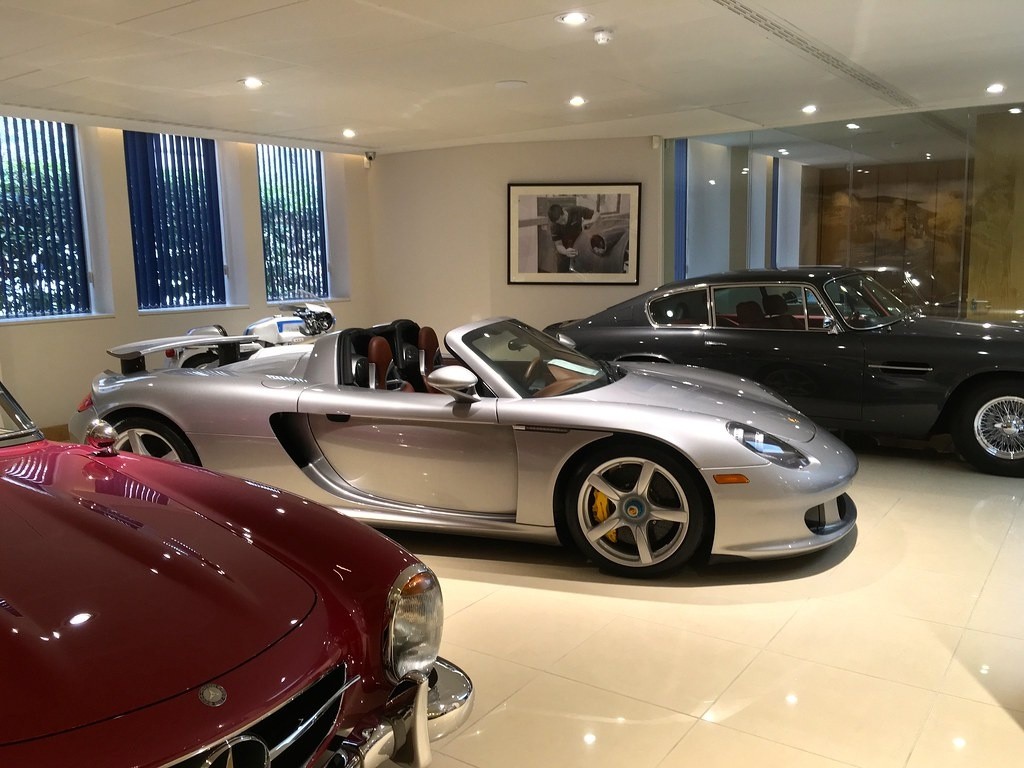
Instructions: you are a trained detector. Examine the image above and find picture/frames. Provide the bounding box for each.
[597,193,621,215]
[507,182,641,285]
[816,158,974,320]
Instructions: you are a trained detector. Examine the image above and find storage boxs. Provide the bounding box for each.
[40,423,76,444]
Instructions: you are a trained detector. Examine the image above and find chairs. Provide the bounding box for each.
[736,302,766,328]
[671,303,698,324]
[369,336,416,393]
[762,295,805,330]
[419,327,446,394]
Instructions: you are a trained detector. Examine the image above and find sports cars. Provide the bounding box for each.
[0,382,445,767]
[535,264,1024,478]
[66,300,861,581]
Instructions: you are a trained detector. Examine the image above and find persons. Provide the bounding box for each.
[548,204,600,273]
